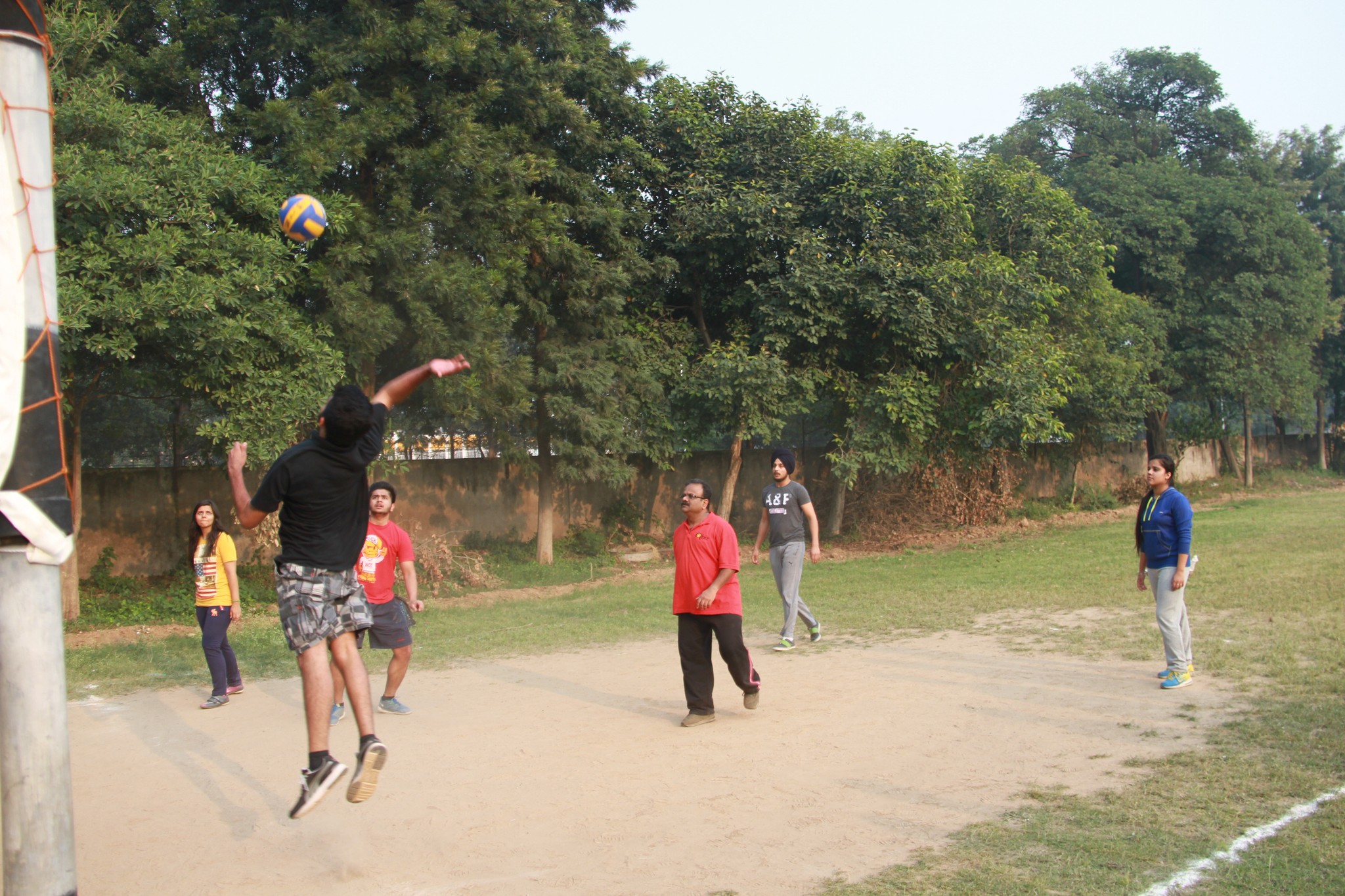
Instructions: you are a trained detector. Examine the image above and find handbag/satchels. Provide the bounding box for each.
[393,594,416,627]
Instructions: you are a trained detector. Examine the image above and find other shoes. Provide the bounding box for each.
[681,713,716,727]
[743,692,759,709]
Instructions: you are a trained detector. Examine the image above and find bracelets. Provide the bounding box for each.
[232,601,239,602]
[1139,571,1146,576]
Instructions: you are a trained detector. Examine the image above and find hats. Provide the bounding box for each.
[770,449,795,474]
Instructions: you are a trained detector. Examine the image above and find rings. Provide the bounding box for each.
[1176,584,1179,586]
[1180,584,1182,586]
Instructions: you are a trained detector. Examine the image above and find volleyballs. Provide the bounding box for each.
[278,192,328,243]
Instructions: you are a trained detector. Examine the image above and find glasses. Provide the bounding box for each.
[680,494,705,500]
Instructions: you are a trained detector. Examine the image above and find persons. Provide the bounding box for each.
[1135,454,1197,690]
[229,355,470,819]
[673,478,760,728]
[328,479,425,725]
[751,449,822,651]
[189,500,244,709]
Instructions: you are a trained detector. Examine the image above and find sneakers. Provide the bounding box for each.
[287,756,347,819]
[1157,663,1194,678]
[808,621,821,642]
[377,697,412,715]
[201,695,230,708]
[226,684,244,695]
[1161,672,1192,689]
[773,638,795,650]
[327,705,345,726]
[345,739,387,803]
